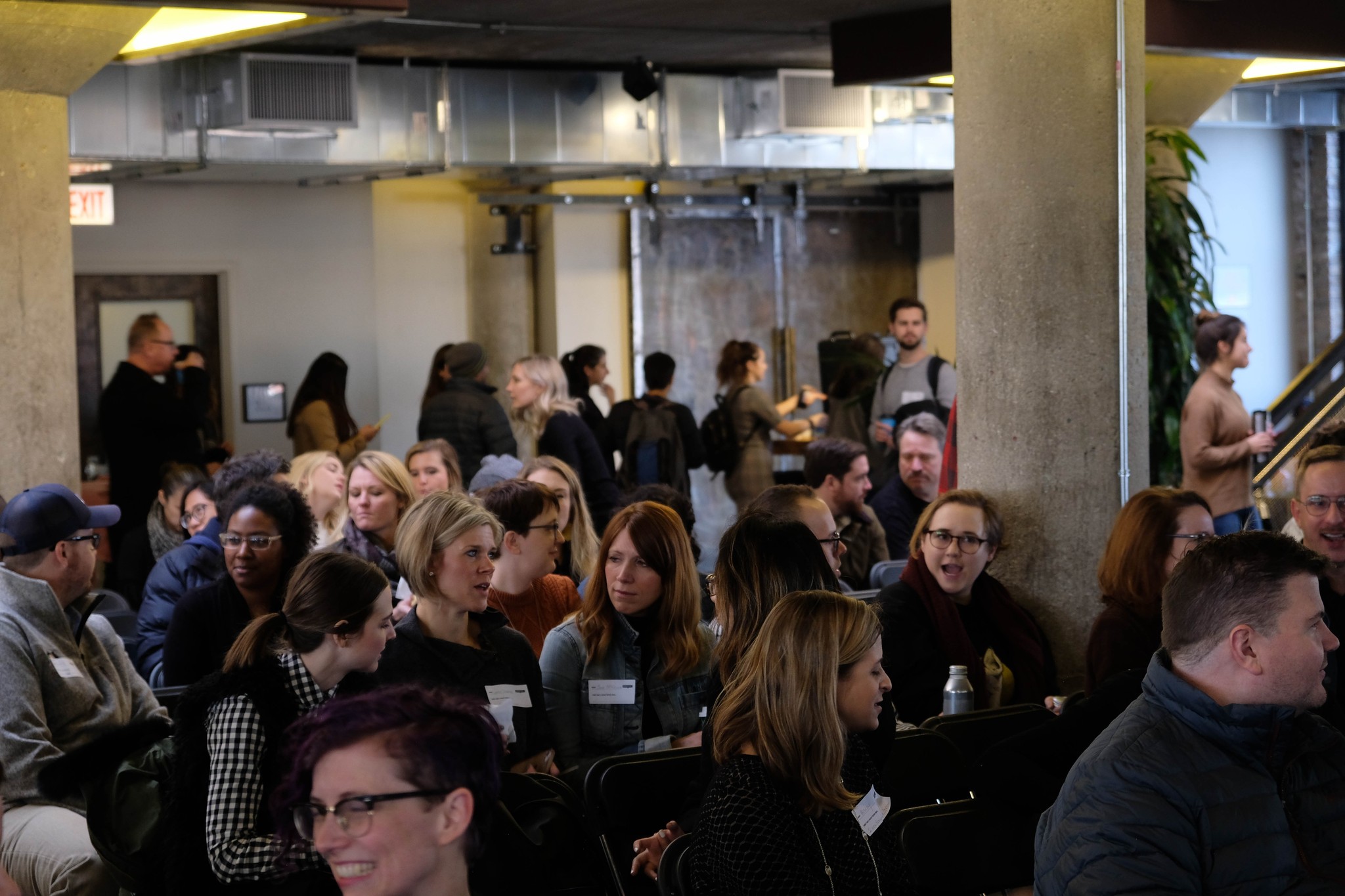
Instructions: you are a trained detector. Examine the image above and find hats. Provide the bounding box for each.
[445,342,488,380]
[1,483,121,557]
[466,453,523,494]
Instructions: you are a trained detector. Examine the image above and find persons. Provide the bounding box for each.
[868,486,1068,729]
[628,507,899,883]
[1031,527,1345,896]
[1287,444,1344,739]
[1178,309,1279,539]
[801,435,891,591]
[536,500,724,794]
[866,294,958,493]
[161,548,399,896]
[87,307,720,706]
[0,482,175,896]
[866,410,947,561]
[740,483,944,732]
[333,488,562,778]
[1084,483,1220,744]
[697,338,830,520]
[283,683,508,896]
[690,588,1035,896]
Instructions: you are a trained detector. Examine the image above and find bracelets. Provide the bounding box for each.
[804,417,815,431]
[795,386,807,410]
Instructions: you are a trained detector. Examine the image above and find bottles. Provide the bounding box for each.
[942,664,977,719]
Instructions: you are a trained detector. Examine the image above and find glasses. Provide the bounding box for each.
[1171,532,1220,547]
[219,532,282,552]
[179,504,211,529]
[704,573,718,597]
[290,791,425,842]
[1297,495,1345,517]
[527,522,562,542]
[148,338,178,350]
[814,531,842,553]
[921,528,990,555]
[48,533,101,553]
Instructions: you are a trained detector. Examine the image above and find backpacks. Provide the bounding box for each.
[698,382,761,479]
[615,397,686,491]
